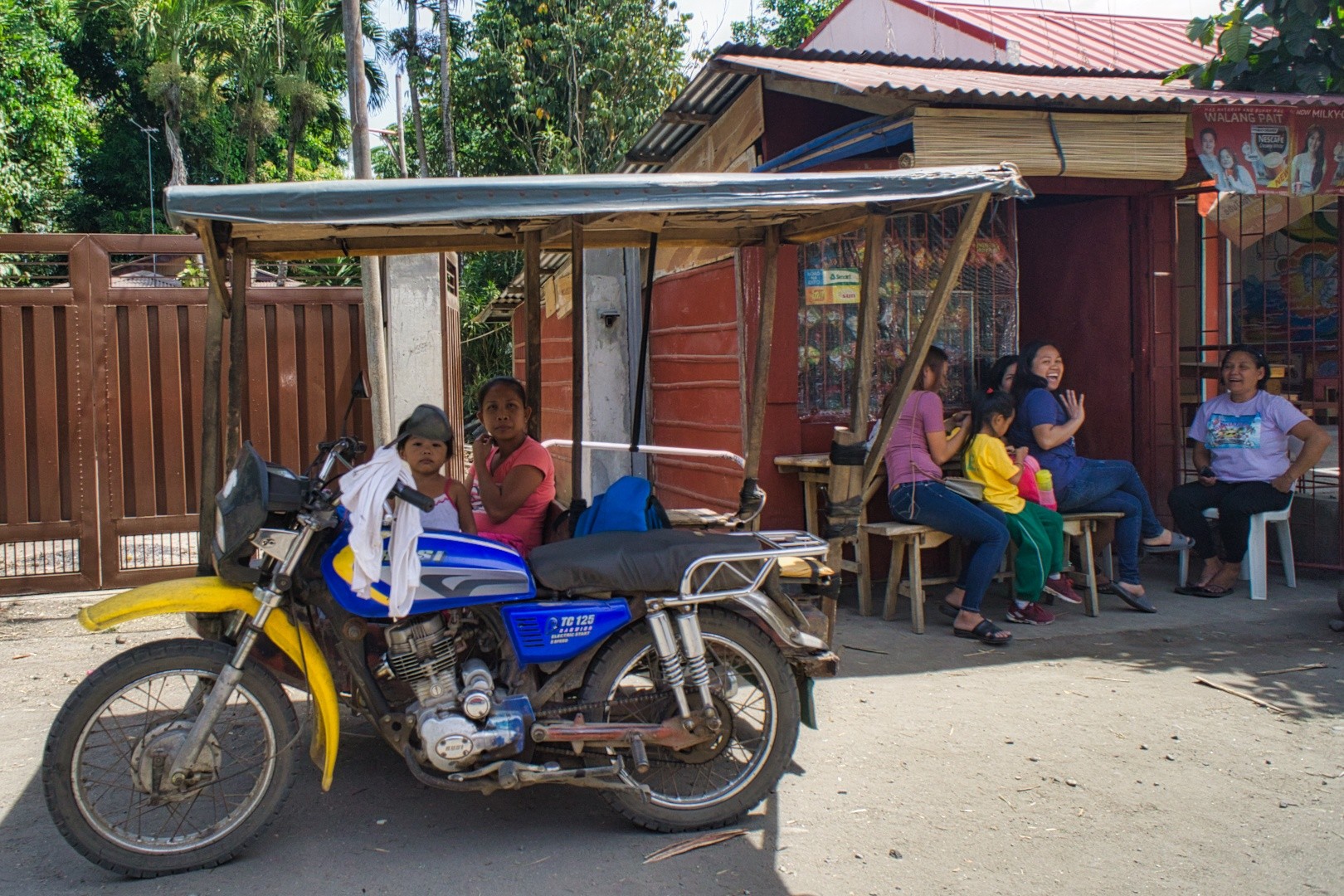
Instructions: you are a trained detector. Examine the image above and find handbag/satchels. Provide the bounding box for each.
[943,477,984,501]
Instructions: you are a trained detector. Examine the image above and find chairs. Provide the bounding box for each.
[1176,478,1299,598]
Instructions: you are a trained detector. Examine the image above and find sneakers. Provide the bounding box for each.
[1043,573,1082,604]
[1006,602,1055,625]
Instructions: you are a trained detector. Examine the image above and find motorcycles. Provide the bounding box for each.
[41,368,842,881]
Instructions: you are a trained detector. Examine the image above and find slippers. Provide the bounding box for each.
[1073,584,1115,594]
[1115,584,1157,612]
[1174,585,1203,595]
[954,619,1013,643]
[1142,532,1195,552]
[939,602,959,618]
[1194,584,1234,598]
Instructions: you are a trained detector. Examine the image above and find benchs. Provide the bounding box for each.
[858,512,1121,633]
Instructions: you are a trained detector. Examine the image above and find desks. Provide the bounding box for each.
[774,453,968,615]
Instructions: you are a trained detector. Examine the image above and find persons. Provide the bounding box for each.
[381,376,555,629]
[1168,346,1329,594]
[865,340,1195,644]
[1198,126,1257,194]
[1290,124,1326,196]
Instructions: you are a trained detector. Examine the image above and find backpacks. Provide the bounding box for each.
[573,476,673,537]
[1010,455,1057,511]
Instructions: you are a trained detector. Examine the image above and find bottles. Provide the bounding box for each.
[1035,469,1053,491]
